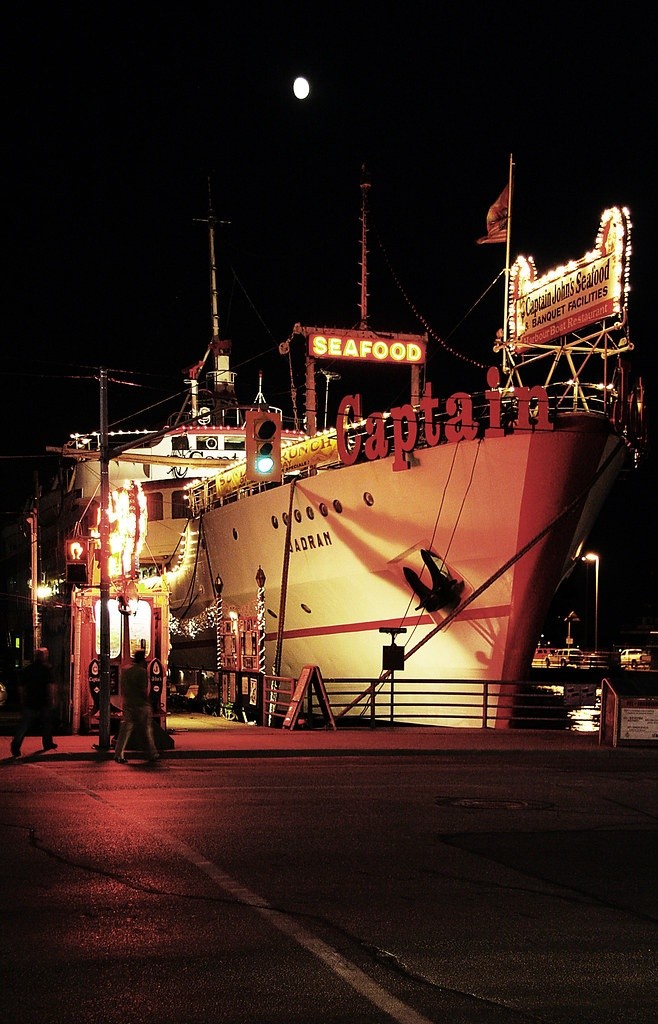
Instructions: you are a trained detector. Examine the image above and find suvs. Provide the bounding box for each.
[545,648,584,668]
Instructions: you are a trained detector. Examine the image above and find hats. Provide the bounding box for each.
[37,647,47,654]
[133,651,146,662]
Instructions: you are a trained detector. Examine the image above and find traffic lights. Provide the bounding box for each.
[246,411,281,482]
[66,539,89,585]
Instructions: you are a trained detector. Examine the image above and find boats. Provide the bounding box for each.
[21,178,655,729]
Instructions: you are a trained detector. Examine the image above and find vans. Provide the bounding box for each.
[533,647,558,663]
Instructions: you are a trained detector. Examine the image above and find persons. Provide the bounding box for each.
[11,647,57,755]
[113,651,166,763]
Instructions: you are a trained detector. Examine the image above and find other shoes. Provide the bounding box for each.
[149,753,159,760]
[115,757,128,764]
[43,743,58,750]
[11,741,21,757]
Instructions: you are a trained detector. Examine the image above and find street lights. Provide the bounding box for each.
[583,553,600,653]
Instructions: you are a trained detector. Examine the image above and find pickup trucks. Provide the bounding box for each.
[619,648,653,670]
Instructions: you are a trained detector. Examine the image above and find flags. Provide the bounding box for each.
[476,174,510,245]
